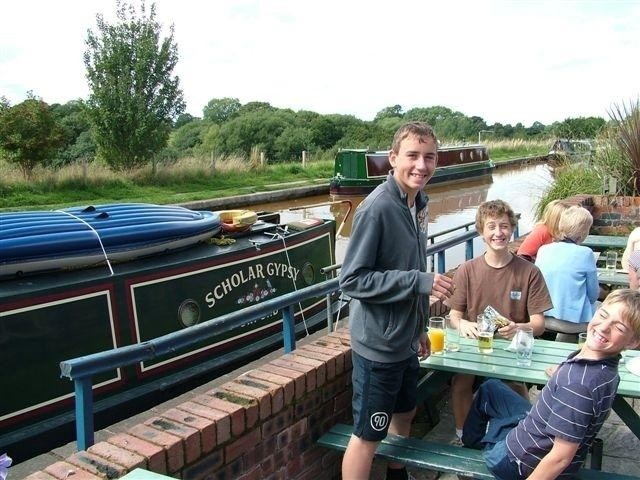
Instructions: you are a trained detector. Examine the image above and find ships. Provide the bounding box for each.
[329,144,496,201]
[0,198,357,459]
[547,139,628,167]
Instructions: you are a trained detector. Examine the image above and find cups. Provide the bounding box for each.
[476,315,493,354]
[516,327,533,367]
[445,314,460,352]
[429,316,444,356]
[606,252,617,277]
[579,333,588,348]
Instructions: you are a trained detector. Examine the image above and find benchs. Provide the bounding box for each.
[315,421,639,480]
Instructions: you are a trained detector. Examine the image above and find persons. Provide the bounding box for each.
[534,205,603,334]
[619,229,640,290]
[461,287,640,480]
[338,121,456,480]
[517,199,572,263]
[448,199,555,449]
